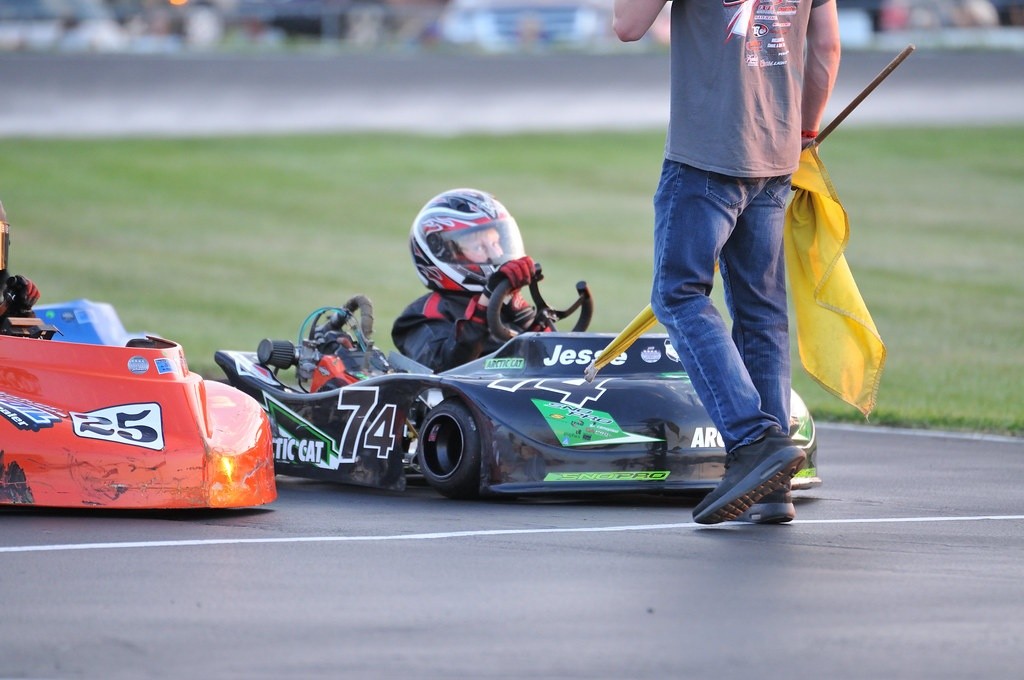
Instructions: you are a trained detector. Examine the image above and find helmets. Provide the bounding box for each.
[408,189,525,293]
[0,201,10,270]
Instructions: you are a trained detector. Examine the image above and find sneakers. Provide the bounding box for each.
[692,425,806,525]
[730,479,795,523]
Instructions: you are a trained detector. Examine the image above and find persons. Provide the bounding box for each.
[0,197,41,336]
[605,0,847,525]
[392,186,555,370]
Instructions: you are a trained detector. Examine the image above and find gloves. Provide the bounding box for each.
[3,275,40,313]
[482,256,535,300]
